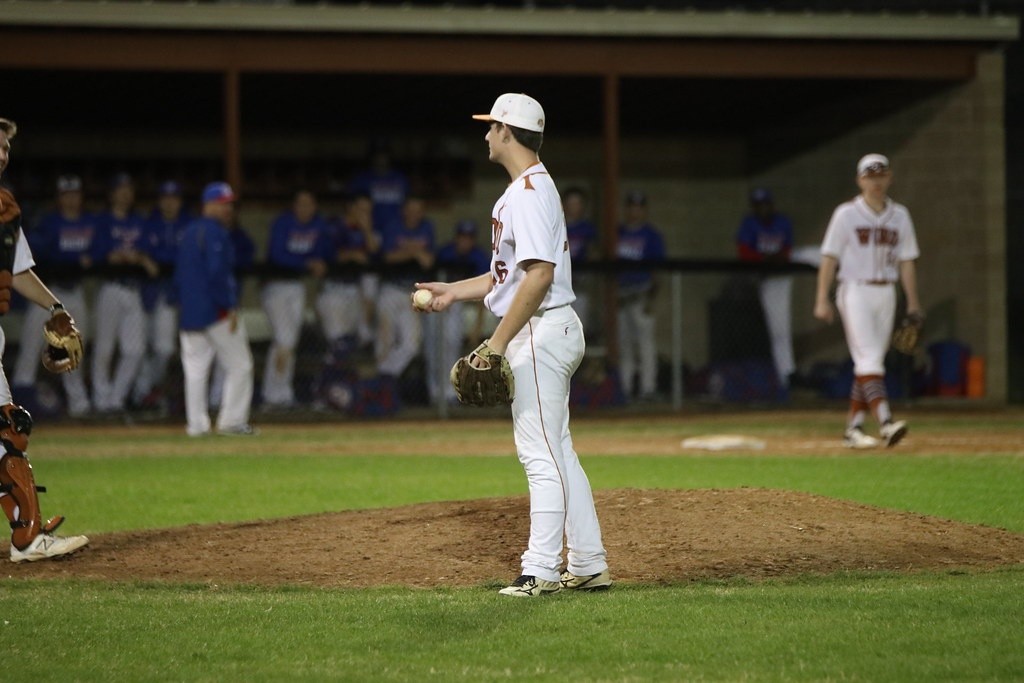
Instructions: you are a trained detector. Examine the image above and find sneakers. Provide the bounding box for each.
[498,575,561,597]
[10,532,89,564]
[559,569,613,591]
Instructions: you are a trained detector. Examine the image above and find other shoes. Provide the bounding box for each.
[880,420,907,447]
[841,426,878,449]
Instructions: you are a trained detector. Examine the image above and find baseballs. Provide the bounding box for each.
[413,288,433,309]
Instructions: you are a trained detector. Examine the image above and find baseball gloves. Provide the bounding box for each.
[42,311,87,374]
[449,338,517,409]
[891,313,925,356]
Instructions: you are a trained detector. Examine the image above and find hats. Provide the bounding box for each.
[857,154,889,180]
[204,184,232,203]
[472,93,545,133]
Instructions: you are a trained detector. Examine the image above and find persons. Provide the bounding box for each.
[814,154,922,451]
[736,187,799,395]
[0,118,89,565]
[10,135,668,437]
[411,93,611,597]
